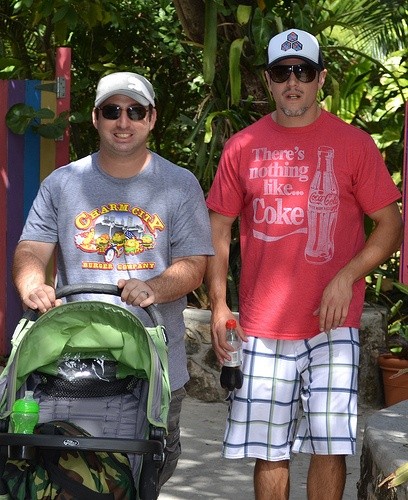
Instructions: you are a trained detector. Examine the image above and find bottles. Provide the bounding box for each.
[219,319,243,391]
[9,391,39,434]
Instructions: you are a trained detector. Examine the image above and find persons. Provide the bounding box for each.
[12,72,215,489]
[205,28,404,500]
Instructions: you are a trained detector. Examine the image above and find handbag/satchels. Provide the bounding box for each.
[3,420,136,500]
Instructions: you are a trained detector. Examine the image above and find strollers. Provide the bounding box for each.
[0,283,172,500]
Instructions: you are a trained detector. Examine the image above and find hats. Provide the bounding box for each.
[94,72,156,108]
[266,29,324,73]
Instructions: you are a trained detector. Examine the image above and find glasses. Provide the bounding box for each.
[98,106,149,121]
[266,63,316,83]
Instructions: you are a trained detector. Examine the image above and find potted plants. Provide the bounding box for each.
[378,281,408,407]
